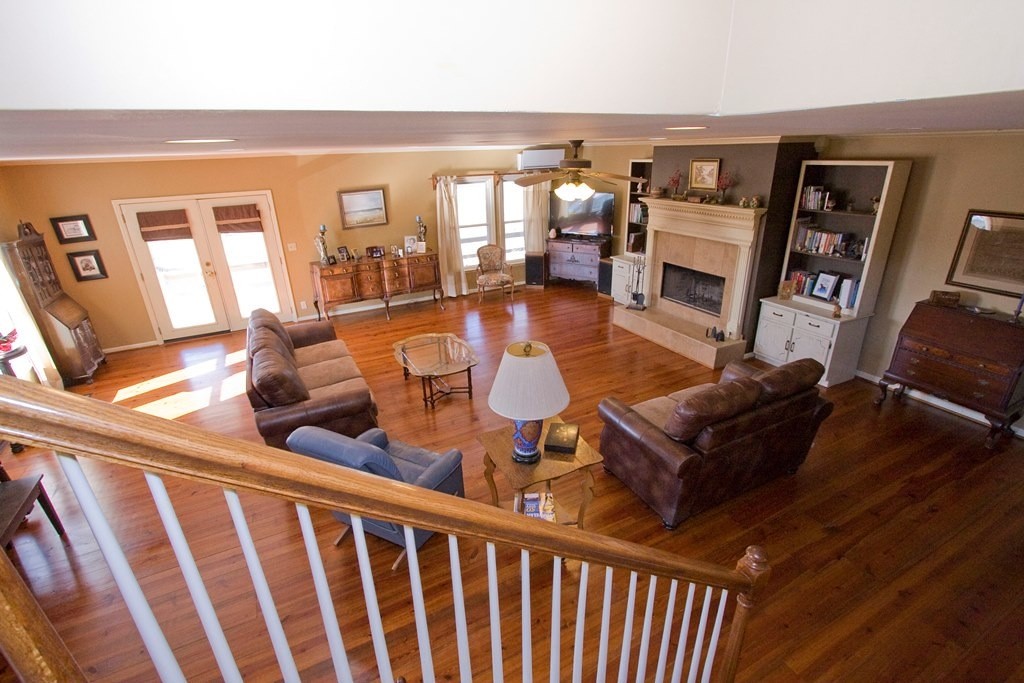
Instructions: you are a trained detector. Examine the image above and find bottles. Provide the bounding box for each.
[739,196,748,208]
[749,195,760,208]
[549,228,556,239]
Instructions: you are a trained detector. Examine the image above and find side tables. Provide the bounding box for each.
[471,416,603,561]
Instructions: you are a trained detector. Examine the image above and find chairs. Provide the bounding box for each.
[0,465,65,549]
[477,244,515,304]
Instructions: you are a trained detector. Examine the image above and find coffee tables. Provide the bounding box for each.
[393,333,478,409]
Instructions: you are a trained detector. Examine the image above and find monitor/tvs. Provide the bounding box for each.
[549,191,616,242]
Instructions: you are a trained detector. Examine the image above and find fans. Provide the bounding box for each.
[514,140,648,187]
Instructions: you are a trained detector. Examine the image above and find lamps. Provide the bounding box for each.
[553,179,596,202]
[486,341,572,467]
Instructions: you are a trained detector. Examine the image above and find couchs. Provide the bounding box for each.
[597,358,833,529]
[242,306,464,570]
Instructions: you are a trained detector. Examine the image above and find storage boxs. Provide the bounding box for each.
[515,491,556,523]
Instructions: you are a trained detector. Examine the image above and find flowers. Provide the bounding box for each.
[716,176,734,189]
[667,176,680,187]
[0,329,18,353]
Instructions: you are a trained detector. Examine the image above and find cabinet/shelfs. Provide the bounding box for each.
[753,161,910,387]
[875,297,1024,449]
[309,248,445,322]
[7,232,108,385]
[542,235,612,292]
[609,160,653,306]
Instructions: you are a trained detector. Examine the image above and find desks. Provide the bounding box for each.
[0,346,29,454]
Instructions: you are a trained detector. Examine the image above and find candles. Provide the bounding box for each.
[416,215,421,222]
[320,224,325,231]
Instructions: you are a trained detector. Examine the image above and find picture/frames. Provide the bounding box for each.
[688,159,719,191]
[327,236,425,265]
[337,188,388,229]
[945,209,1024,300]
[49,214,108,282]
[778,270,840,302]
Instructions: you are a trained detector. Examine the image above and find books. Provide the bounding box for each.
[797,183,838,213]
[791,216,871,263]
[629,203,648,223]
[786,268,818,296]
[512,492,557,527]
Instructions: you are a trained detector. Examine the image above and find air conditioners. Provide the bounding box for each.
[516,149,566,172]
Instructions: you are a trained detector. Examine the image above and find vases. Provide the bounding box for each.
[718,189,728,204]
[671,187,678,199]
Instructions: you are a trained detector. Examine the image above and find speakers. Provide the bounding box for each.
[597,258,613,301]
[525,251,548,290]
